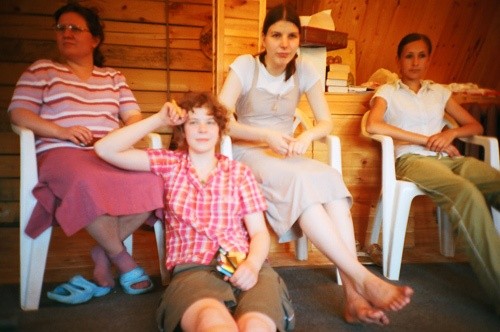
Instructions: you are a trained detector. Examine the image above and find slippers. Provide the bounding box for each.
[119,266,154,294]
[47,274,114,304]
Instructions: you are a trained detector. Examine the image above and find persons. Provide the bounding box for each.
[94,93,295,332]
[366,33,500,300]
[216,4,414,325]
[7,4,163,294]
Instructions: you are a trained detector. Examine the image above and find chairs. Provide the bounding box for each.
[11,121,170,311]
[361,108,500,281]
[219,107,344,287]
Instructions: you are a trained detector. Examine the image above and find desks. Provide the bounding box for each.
[297,93,500,253]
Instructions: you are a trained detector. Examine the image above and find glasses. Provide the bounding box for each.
[56,24,90,33]
